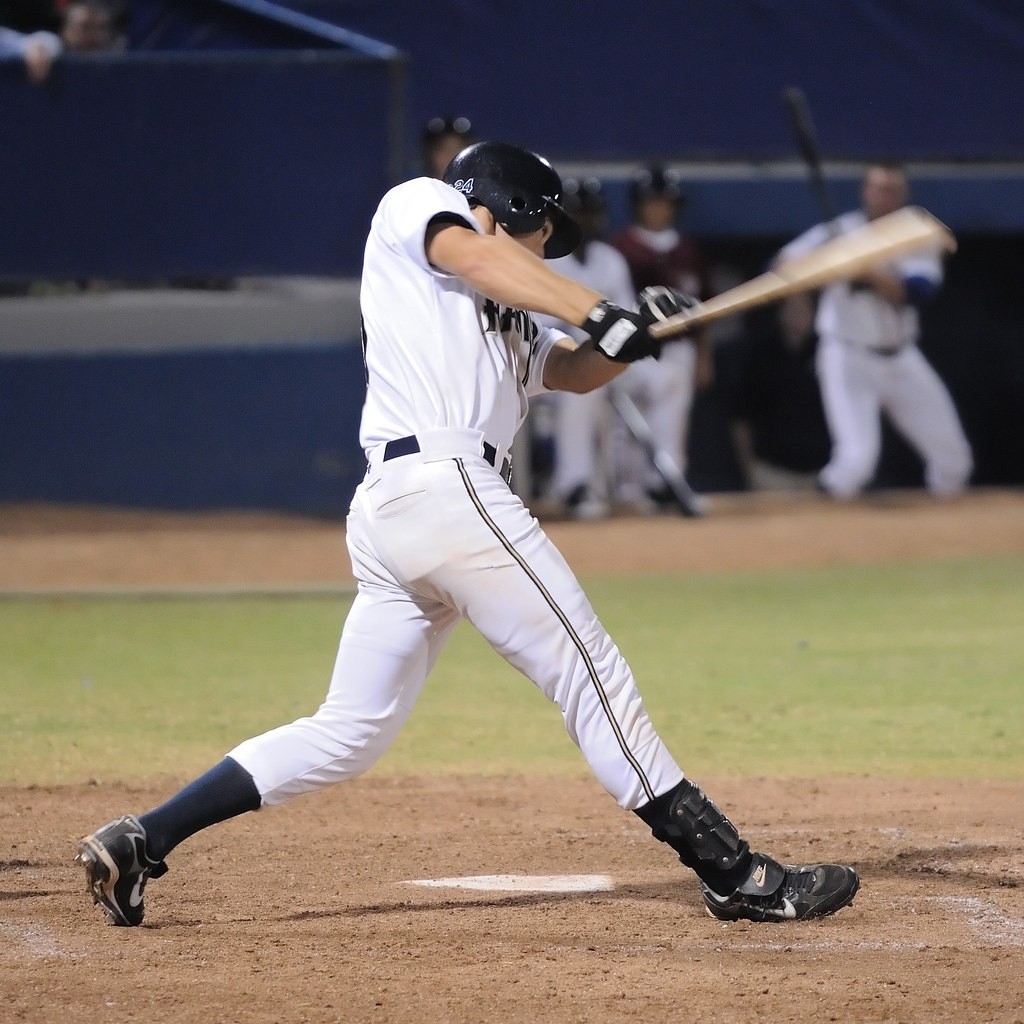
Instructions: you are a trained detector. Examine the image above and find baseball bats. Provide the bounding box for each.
[644,211,957,332]
[788,86,841,240]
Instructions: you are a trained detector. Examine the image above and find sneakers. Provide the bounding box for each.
[74,814,168,926]
[702,852,860,923]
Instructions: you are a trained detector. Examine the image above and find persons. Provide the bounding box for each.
[0,0,117,85]
[75,140,861,928]
[426,118,977,521]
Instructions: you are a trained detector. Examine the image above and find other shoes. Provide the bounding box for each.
[646,479,677,508]
[567,484,609,519]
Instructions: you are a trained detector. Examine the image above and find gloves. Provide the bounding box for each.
[581,300,661,363]
[640,293,700,322]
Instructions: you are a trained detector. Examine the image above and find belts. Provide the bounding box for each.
[384,435,513,485]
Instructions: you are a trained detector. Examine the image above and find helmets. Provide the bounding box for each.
[423,114,474,149]
[631,168,687,207]
[442,140,584,259]
[561,176,606,214]
